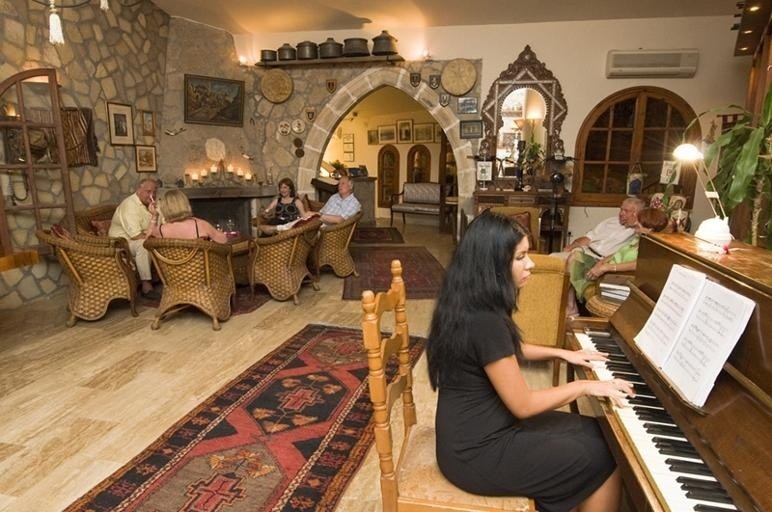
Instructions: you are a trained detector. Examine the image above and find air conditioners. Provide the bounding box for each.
[609,50,698,77]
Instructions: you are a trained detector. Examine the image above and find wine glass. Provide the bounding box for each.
[226,219,235,231]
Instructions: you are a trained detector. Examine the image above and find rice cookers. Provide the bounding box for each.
[296,40,319,59]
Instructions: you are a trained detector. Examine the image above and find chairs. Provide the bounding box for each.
[490,205,542,255]
[34,226,140,329]
[248,218,323,306]
[144,233,234,331]
[359,260,538,512]
[248,194,315,236]
[312,201,364,277]
[511,255,569,387]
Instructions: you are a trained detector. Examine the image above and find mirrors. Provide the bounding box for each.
[482,46,567,189]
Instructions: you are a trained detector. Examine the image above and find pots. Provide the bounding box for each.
[278,43,296,60]
[319,37,343,58]
[261,49,277,61]
[344,37,369,56]
[372,30,399,54]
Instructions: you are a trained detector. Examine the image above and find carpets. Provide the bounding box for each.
[342,245,452,300]
[51,322,428,511]
[138,284,272,315]
[349,227,405,244]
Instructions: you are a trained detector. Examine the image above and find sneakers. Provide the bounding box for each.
[141,288,161,301]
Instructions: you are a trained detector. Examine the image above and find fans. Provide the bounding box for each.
[533,155,580,253]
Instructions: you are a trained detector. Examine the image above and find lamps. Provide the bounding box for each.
[673,143,736,246]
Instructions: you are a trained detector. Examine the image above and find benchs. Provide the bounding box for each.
[390,182,444,232]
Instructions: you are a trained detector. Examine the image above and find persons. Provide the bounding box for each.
[109,180,162,301]
[146,190,227,244]
[427,210,636,512]
[549,198,648,260]
[259,175,358,241]
[566,208,668,317]
[261,177,306,236]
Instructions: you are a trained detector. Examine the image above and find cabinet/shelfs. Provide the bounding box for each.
[474,190,569,252]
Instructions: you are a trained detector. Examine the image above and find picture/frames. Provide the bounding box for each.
[107,102,134,145]
[414,124,434,143]
[142,110,155,137]
[378,125,397,144]
[434,124,449,143]
[136,146,156,172]
[367,130,379,143]
[184,73,244,128]
[458,97,477,113]
[397,120,412,144]
[460,120,483,139]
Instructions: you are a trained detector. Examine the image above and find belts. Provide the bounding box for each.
[587,245,604,258]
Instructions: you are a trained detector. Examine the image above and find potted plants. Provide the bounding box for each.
[521,143,541,189]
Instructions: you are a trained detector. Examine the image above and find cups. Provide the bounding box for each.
[215,223,223,233]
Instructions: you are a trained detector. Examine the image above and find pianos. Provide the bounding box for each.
[564,229,768,512]
